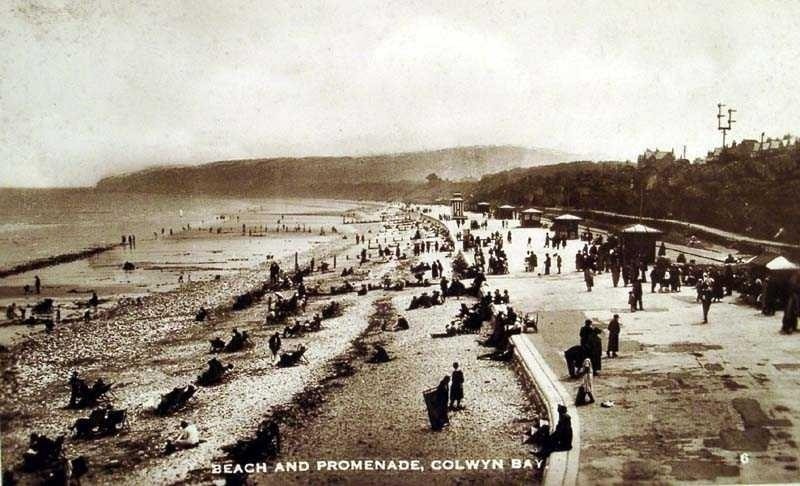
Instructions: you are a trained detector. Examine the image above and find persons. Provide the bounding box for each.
[524,231,800,334]
[2,198,516,485]
[563,319,602,378]
[529,404,573,458]
[607,315,621,360]
[581,358,595,403]
